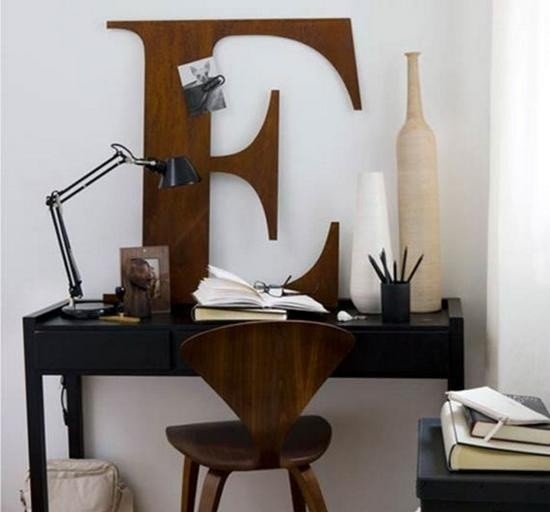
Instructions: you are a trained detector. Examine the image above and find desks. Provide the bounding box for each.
[16,291,467,512]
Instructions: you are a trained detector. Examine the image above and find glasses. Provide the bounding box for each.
[254,275,316,298]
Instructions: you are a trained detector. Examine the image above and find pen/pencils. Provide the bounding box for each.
[369,247,424,284]
[98,316,140,323]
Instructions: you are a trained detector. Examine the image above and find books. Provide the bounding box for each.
[442,384,549,473]
[191,265,329,326]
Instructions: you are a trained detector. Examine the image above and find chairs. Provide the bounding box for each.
[162,320,357,511]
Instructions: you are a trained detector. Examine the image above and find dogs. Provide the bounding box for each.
[188,60,211,88]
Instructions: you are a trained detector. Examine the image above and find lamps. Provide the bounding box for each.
[39,138,202,319]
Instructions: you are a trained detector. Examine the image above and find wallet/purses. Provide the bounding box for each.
[447,387,550,425]
